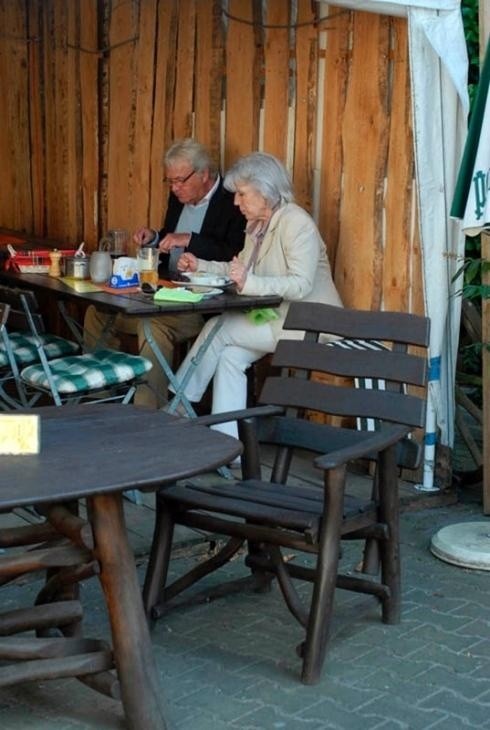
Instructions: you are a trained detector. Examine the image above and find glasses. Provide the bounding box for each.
[163,170,195,184]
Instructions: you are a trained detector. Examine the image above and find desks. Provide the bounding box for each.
[2,263,284,509]
[1,388,244,730]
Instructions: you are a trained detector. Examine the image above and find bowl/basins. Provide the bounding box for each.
[60,255,92,282]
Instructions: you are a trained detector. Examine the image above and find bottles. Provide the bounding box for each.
[47,248,61,277]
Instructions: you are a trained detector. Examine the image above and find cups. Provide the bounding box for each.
[136,247,161,290]
[90,250,111,283]
[107,227,127,258]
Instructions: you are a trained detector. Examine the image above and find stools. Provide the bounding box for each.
[280,342,400,438]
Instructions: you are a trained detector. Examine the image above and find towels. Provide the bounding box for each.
[242,305,277,324]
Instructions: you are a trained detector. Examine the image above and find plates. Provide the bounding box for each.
[172,272,235,288]
[176,286,223,301]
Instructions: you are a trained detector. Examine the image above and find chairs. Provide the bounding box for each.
[1,279,156,506]
[141,300,431,685]
[2,307,90,411]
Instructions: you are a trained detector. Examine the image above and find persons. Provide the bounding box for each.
[81,135,245,407]
[159,151,347,470]
